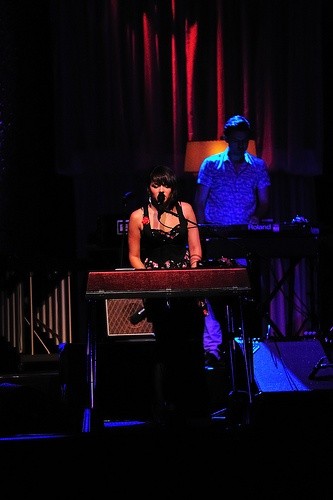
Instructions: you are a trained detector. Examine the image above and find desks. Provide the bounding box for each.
[85,287,253,408]
[119,231,322,329]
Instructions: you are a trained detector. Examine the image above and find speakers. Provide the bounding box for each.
[104,296,156,337]
[251,337,333,395]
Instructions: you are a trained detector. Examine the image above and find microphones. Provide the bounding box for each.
[308,356,324,380]
[157,190,166,208]
[130,306,145,324]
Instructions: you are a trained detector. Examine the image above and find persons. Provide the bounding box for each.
[128,162,216,419]
[193,114,273,349]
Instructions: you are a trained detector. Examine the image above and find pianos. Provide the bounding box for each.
[197,216,320,337]
[85,259,253,407]
